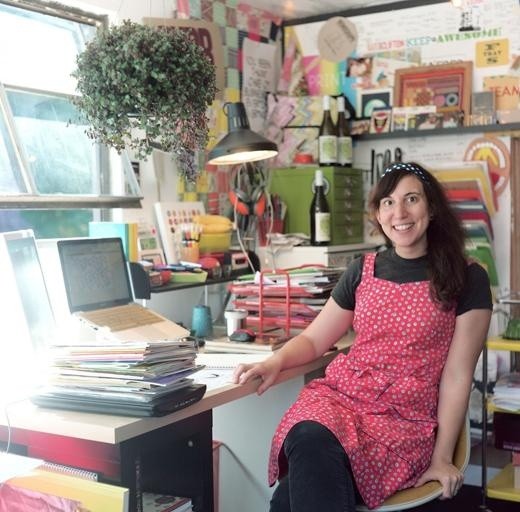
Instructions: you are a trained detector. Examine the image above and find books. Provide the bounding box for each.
[1,451,193,511]
[34,337,208,417]
[491,293,519,470]
[88,199,209,284]
[194,263,347,369]
[427,162,501,289]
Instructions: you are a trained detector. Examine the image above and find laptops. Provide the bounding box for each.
[57,237,191,342]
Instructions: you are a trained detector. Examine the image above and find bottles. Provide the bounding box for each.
[192,304,212,337]
[335,95,353,168]
[309,169,332,246]
[318,95,338,167]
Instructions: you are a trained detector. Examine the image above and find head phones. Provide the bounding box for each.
[229,165,266,217]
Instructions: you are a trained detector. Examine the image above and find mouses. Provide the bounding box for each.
[229,329,255,342]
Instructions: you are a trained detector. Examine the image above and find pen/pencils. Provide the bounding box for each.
[181,223,203,249]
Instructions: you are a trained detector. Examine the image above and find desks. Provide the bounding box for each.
[0,316,353,511]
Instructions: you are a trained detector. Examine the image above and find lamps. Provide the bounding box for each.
[207,101,279,182]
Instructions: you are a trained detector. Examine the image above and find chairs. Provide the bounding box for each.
[354,411,471,511]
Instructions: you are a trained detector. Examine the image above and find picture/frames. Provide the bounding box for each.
[393,62,471,125]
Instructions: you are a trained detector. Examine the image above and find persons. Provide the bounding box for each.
[233,162,494,510]
[415,111,463,130]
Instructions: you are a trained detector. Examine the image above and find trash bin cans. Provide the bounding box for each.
[212,440,222,512]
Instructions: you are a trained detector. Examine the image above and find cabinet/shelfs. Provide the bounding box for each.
[271,166,365,244]
[481,293,520,512]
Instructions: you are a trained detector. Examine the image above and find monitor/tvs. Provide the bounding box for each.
[0,229,58,346]
